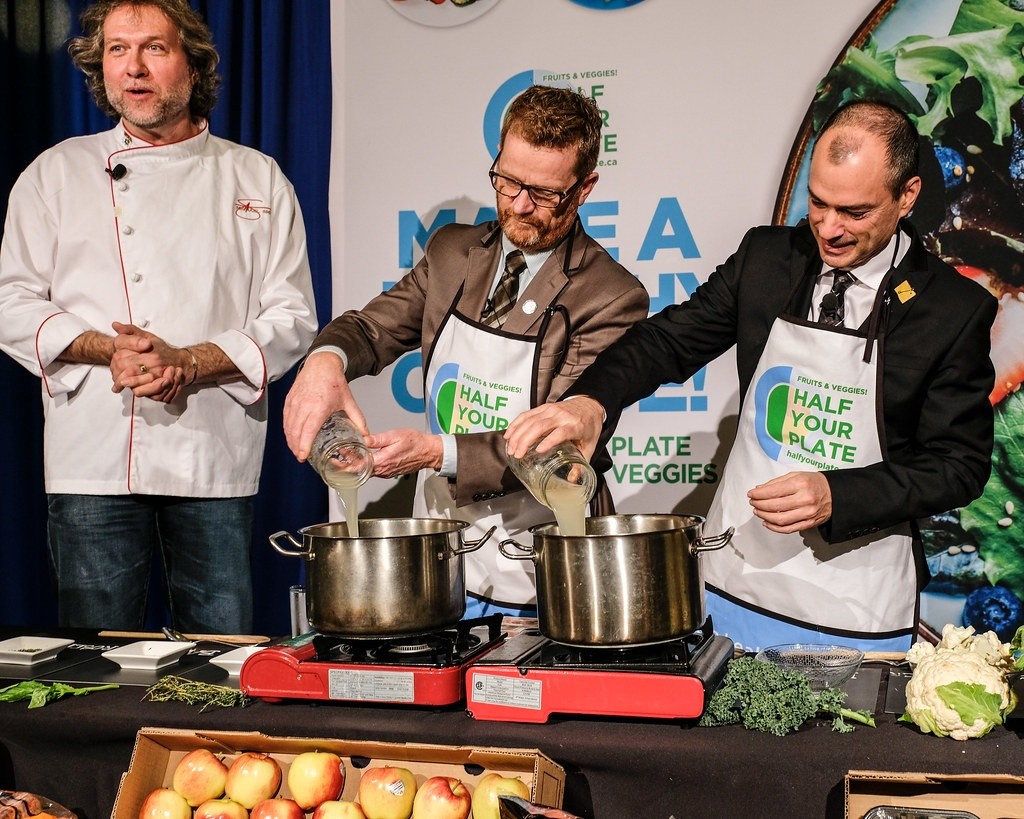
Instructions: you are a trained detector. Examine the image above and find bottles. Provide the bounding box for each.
[307,410,374,490]
[505,432,597,510]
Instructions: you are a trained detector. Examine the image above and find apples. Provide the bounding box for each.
[137,749,529,819]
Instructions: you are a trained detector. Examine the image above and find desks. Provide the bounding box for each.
[0,630,1024,819]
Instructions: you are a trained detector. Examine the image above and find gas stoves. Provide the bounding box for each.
[240,625,484,707]
[465,628,735,723]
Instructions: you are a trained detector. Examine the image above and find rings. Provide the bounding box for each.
[140,364,147,372]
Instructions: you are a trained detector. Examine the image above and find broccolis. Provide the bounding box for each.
[905,623,1023,742]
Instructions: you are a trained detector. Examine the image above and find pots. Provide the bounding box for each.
[498,509,735,651]
[269,518,497,641]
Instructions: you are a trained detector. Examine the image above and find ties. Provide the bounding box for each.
[479,247,527,330]
[817,270,860,329]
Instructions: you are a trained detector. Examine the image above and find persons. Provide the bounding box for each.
[0,0,318,637]
[282,85,650,621]
[503,99,998,660]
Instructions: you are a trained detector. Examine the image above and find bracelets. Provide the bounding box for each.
[183,348,198,387]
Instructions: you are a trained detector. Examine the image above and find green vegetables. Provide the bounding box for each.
[910,386,1024,604]
[0,680,120,709]
[699,656,876,735]
[140,675,245,713]
[813,0,1024,147]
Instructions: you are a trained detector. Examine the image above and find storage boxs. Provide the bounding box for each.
[109,726,567,819]
[844,769,1024,819]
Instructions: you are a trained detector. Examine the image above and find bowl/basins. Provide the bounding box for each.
[100,641,197,669]
[0,636,75,665]
[754,644,864,699]
[209,647,267,677]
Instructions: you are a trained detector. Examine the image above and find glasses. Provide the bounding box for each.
[489,146,585,207]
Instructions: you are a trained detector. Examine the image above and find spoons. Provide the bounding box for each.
[162,626,239,648]
